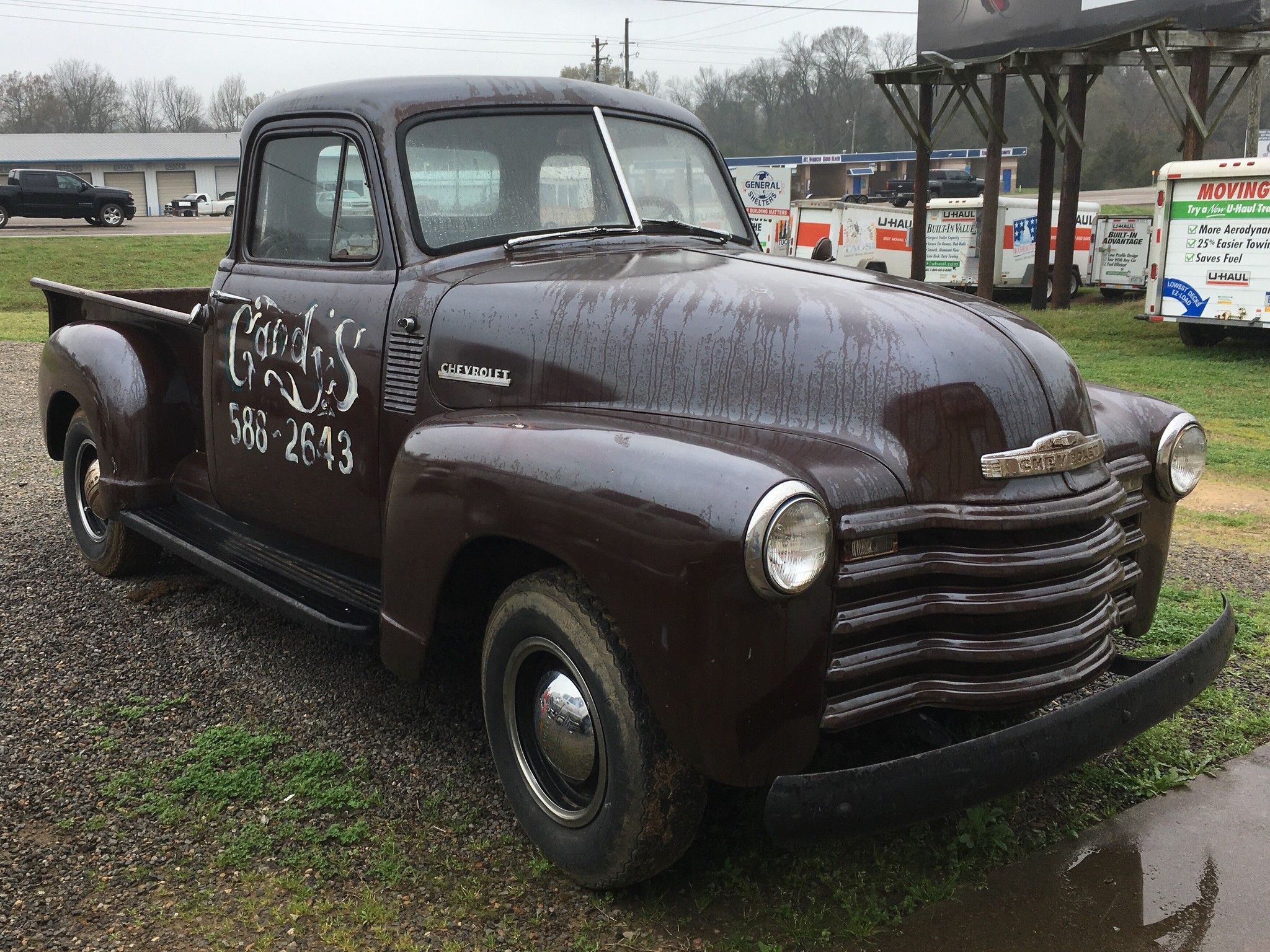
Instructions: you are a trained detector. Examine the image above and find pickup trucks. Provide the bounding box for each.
[28,75,1239,892]
[163,193,235,217]
[886,169,985,208]
[0,168,136,229]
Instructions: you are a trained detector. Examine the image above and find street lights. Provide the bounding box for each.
[846,119,856,154]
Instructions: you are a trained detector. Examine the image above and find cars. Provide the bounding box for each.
[316,190,370,215]
